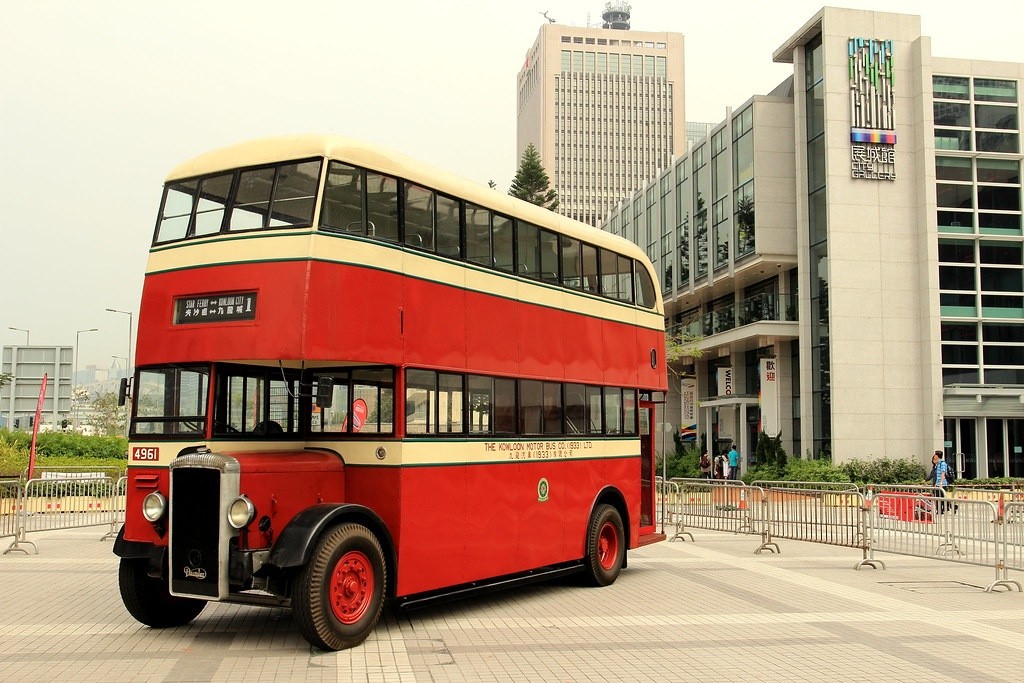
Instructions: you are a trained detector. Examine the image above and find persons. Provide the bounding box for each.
[699,445,739,484]
[924,450,957,515]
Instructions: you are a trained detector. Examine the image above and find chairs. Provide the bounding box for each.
[346,218,632,304]
[252,421,283,432]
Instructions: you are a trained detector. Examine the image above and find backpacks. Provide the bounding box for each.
[701,456,709,469]
[718,457,724,466]
[946,465,955,485]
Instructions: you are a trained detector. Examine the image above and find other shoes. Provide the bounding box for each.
[937,505,958,517]
[700,480,736,486]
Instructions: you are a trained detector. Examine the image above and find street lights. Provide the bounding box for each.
[106,309,132,436]
[75,328,98,386]
[112,355,127,378]
[9,327,29,346]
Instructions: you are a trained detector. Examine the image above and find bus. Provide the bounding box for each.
[112,134,668,654]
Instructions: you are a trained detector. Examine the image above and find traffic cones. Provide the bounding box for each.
[860,487,873,511]
[738,484,748,511]
[992,493,1004,523]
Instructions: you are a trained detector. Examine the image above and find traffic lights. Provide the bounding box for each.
[30,417,33,426]
[62,420,67,428]
[14,419,19,428]
